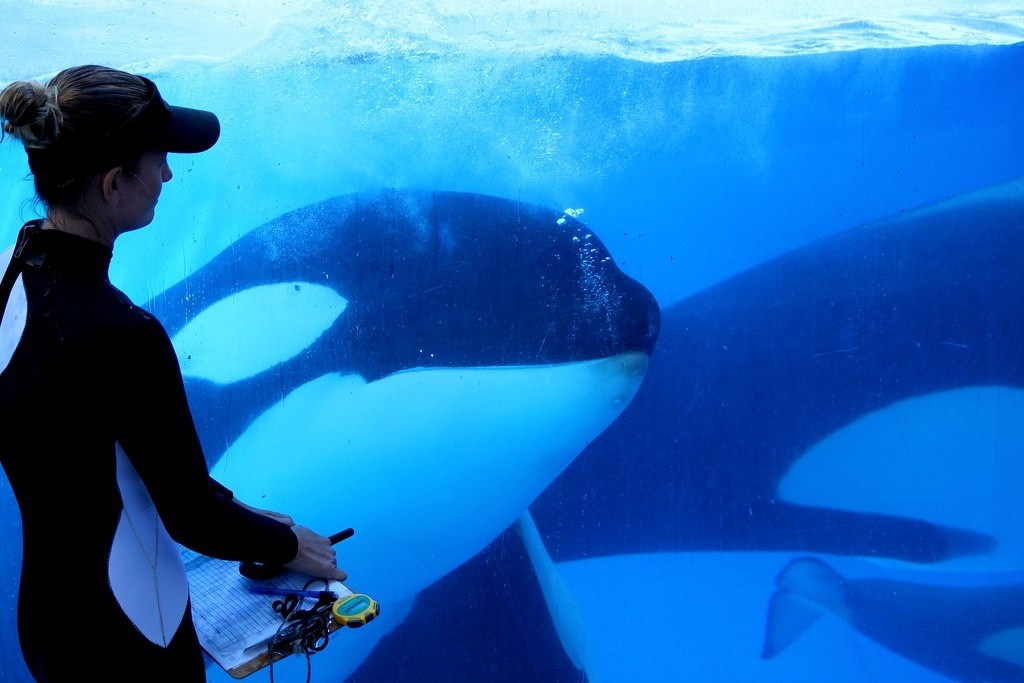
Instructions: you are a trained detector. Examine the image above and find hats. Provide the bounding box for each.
[28,76,220,174]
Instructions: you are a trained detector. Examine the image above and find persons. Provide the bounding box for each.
[0,65,347,683]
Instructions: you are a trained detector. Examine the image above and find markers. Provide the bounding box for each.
[329,529,355,544]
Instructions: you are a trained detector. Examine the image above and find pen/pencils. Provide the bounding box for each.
[247,586,339,600]
